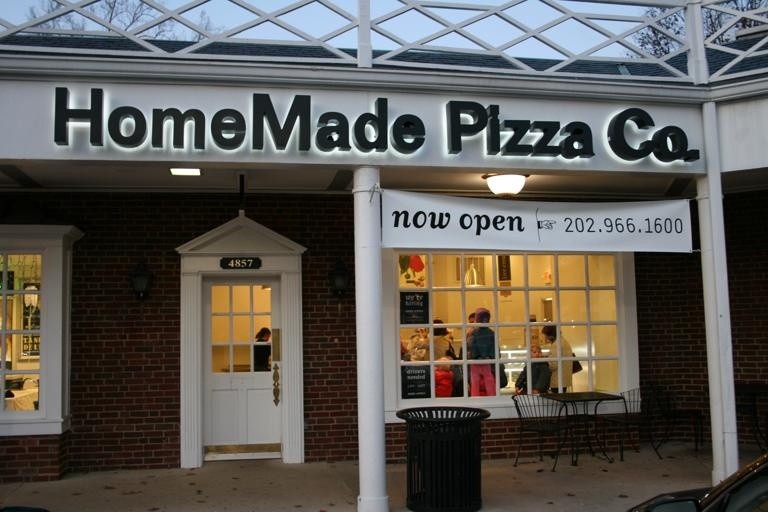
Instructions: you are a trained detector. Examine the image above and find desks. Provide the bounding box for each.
[734,384,765,452]
[539,390,623,470]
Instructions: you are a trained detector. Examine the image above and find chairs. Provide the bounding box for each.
[511,391,575,466]
[603,385,704,458]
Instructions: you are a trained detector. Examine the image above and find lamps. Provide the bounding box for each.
[481,173,530,198]
[327,257,354,298]
[128,259,155,299]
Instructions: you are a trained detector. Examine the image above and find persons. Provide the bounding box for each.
[540,325,573,395]
[424,308,497,394]
[253,326,271,370]
[514,345,551,396]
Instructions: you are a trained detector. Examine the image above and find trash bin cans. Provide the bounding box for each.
[396,406,491,512]
